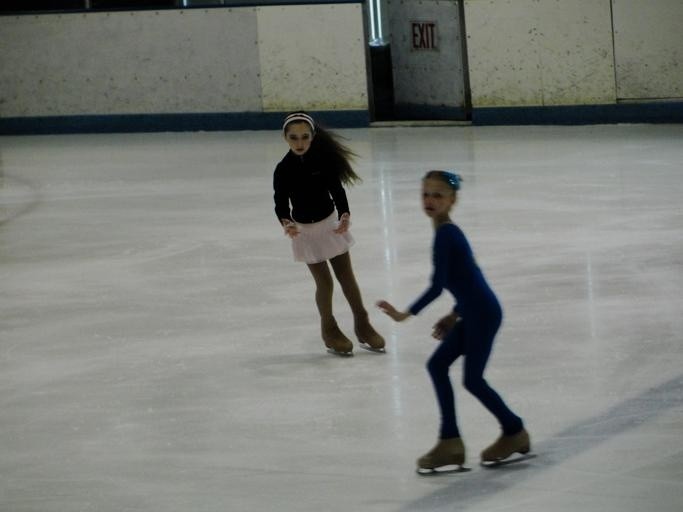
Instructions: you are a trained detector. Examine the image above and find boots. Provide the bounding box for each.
[353,311,385,348]
[320,315,352,352]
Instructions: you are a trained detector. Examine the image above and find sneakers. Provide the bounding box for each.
[417,437,464,469]
[482,428,530,461]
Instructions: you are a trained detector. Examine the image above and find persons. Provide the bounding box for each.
[373,168,540,471]
[270,108,390,353]
